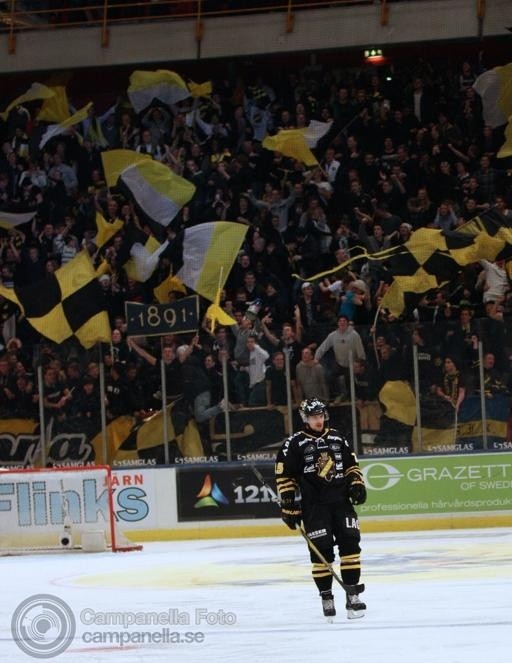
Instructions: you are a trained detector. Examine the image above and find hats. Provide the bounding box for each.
[349,279,366,292]
[301,282,314,290]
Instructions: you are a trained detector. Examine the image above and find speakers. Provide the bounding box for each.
[81,529,107,553]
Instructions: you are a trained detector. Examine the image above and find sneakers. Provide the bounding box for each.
[346,592,366,610]
[319,589,336,616]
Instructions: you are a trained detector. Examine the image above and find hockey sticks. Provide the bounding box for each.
[248,461,365,595]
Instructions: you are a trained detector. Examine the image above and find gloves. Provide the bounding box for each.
[350,484,366,505]
[281,505,301,530]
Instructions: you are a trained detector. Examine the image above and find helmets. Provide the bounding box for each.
[299,396,330,424]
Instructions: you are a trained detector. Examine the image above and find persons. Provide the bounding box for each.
[0,44,511,424]
[275,397,367,617]
